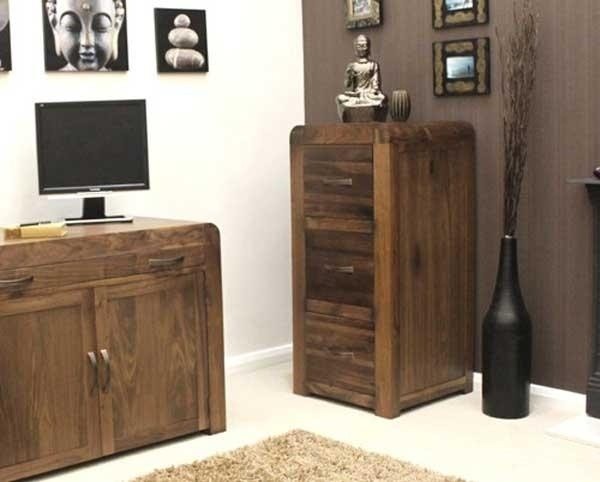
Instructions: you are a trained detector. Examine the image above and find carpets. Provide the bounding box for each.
[123,425,469,481]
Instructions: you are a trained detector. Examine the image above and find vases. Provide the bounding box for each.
[480,234,534,418]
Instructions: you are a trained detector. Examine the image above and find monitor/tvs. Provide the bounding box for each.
[34,99,150,225]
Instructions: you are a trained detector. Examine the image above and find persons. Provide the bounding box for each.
[335,34,386,103]
[46,0,124,72]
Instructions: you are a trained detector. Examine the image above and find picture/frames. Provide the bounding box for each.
[430,0,490,29]
[433,37,491,96]
[343,0,381,30]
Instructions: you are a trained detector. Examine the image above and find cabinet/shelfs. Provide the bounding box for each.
[287,120,476,420]
[0,215,226,479]
